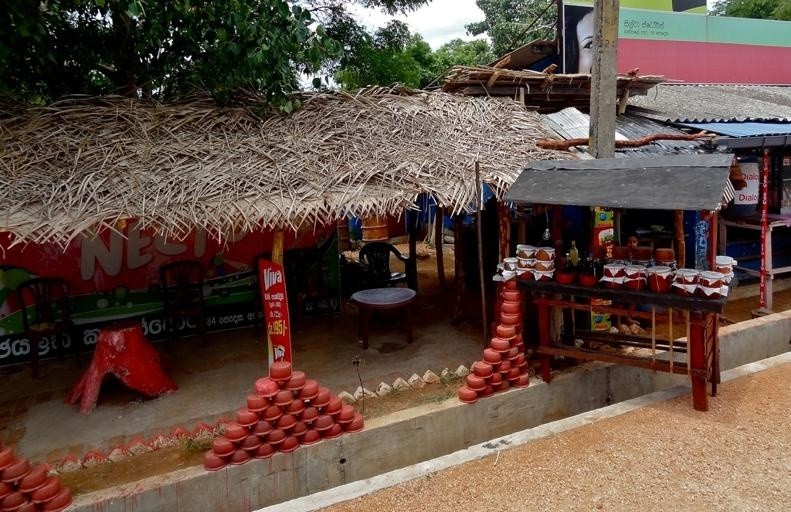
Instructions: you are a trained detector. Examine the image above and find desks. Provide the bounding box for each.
[516,275,740,412]
[349,286,418,350]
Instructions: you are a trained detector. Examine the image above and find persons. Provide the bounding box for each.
[575,9,595,75]
[614,231,641,334]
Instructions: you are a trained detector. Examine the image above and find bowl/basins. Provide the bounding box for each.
[578,272,600,288]
[555,270,576,284]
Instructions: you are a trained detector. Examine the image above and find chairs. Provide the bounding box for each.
[358,240,413,291]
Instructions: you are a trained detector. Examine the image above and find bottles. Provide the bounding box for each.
[566,241,602,278]
